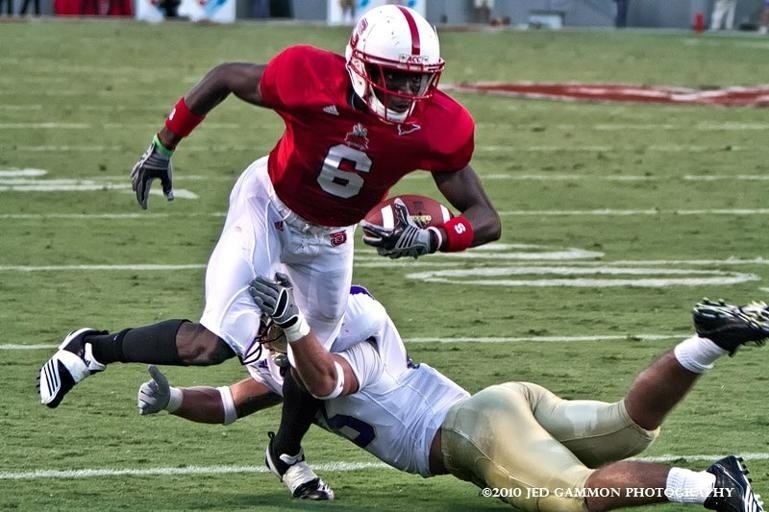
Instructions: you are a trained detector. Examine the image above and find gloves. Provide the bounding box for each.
[137,364,171,416]
[250,272,302,335]
[130,134,175,209]
[358,197,442,259]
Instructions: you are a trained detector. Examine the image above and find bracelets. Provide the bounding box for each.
[424,226,443,251]
[153,133,173,157]
[284,315,311,343]
[166,384,184,415]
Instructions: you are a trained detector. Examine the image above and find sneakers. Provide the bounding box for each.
[692,297,769,356]
[36,327,111,409]
[703,455,767,512]
[265,429,334,501]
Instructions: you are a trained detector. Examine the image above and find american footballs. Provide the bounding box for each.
[364,195,455,238]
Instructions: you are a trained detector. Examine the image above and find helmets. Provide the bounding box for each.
[343,5,445,129]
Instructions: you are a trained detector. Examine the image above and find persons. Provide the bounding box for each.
[136,283,769,511]
[34,4,503,502]
